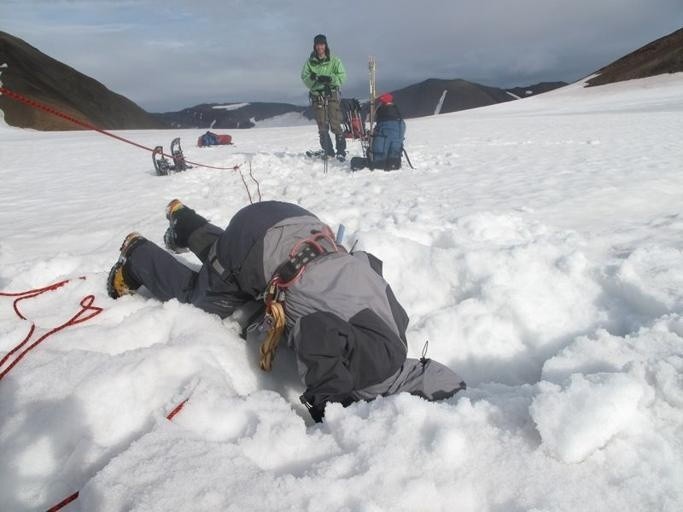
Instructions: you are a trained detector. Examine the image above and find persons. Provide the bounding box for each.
[301,34,346,161]
[108,199,467,421]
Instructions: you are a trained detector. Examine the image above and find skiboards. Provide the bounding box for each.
[368,61,376,170]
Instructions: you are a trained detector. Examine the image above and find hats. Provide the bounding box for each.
[314,35,326,43]
[375,93,394,104]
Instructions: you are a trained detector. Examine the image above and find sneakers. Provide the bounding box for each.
[107,231,144,299]
[163,198,186,250]
[317,149,346,163]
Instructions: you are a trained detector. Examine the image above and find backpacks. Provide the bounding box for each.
[198,131,232,146]
[366,115,406,172]
[339,98,365,138]
